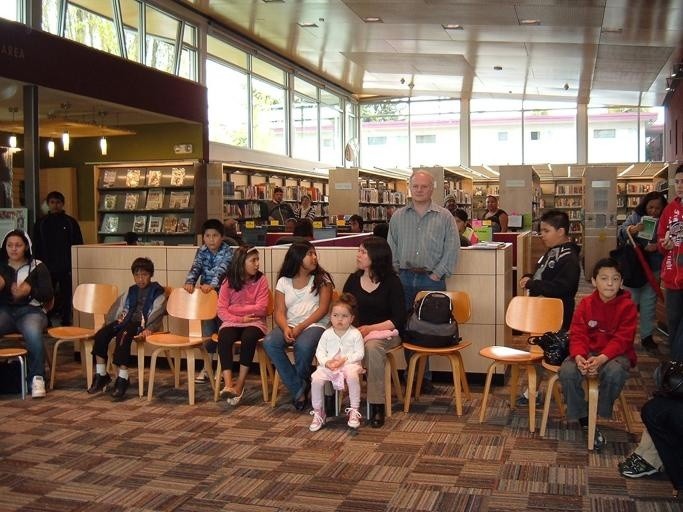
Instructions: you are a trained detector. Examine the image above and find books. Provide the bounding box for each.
[221,180,327,226]
[531,181,585,240]
[637,215,658,240]
[444,177,500,223]
[469,219,507,250]
[96,165,194,245]
[615,181,655,227]
[357,186,407,232]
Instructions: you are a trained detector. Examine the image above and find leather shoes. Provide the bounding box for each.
[227,387,244,406]
[296,400,305,411]
[220,387,236,399]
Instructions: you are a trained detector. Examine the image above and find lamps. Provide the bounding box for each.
[6,105,18,149]
[60,102,70,151]
[98,110,108,156]
[671,62,682,76]
[663,77,681,91]
[46,110,57,158]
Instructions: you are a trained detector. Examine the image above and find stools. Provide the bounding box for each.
[365,342,403,417]
[332,368,368,419]
[538,351,633,450]
[0,348,29,400]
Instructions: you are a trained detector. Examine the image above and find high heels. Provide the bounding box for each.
[372,404,385,428]
[325,396,335,417]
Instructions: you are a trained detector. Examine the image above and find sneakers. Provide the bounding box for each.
[195,369,210,384]
[309,408,326,432]
[618,452,641,469]
[623,457,658,478]
[88,372,112,394]
[31,375,46,398]
[583,425,606,450]
[345,408,362,429]
[515,392,541,406]
[111,376,130,398]
[642,336,656,348]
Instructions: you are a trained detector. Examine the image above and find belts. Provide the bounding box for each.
[406,267,426,273]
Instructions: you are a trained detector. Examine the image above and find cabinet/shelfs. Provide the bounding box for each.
[652,164,682,202]
[329,165,580,232]
[93,160,204,246]
[617,177,656,226]
[206,161,329,231]
[581,165,617,280]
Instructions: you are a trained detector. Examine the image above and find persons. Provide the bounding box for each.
[303,290,365,433]
[451,209,479,244]
[85,256,166,397]
[383,170,461,393]
[481,195,508,234]
[556,256,640,451]
[182,219,236,385]
[215,243,270,407]
[611,190,669,356]
[27,190,86,329]
[372,223,389,240]
[611,361,683,479]
[0,229,54,399]
[347,215,363,233]
[220,215,317,248]
[516,207,584,409]
[341,235,407,430]
[258,239,335,412]
[653,163,683,369]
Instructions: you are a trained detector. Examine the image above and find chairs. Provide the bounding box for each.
[478,296,564,434]
[0,293,54,373]
[402,290,472,419]
[145,286,218,405]
[103,286,172,397]
[210,287,275,402]
[47,283,118,391]
[270,289,342,407]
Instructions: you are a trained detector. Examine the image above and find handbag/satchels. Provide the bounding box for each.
[609,229,647,288]
[536,330,569,366]
[404,292,460,348]
[662,360,683,393]
